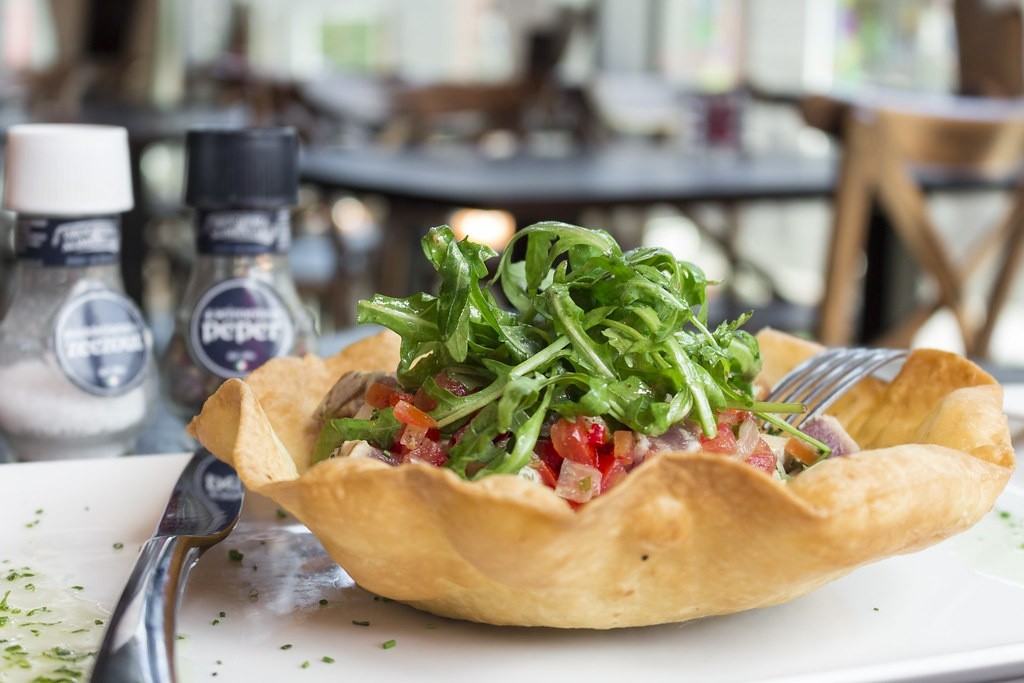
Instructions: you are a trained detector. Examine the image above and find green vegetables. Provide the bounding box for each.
[313,220,830,492]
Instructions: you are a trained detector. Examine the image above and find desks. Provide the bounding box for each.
[0,108,248,328]
[297,142,1024,307]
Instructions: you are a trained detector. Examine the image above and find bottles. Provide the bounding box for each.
[0,121,161,458]
[166,128,315,426]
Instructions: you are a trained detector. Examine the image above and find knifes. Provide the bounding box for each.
[88,435,243,683]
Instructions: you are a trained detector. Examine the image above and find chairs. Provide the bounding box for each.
[384,69,1024,360]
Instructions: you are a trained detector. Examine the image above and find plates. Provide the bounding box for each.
[0,456,1024,683]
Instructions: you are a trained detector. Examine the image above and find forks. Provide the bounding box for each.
[763,350,1024,426]
